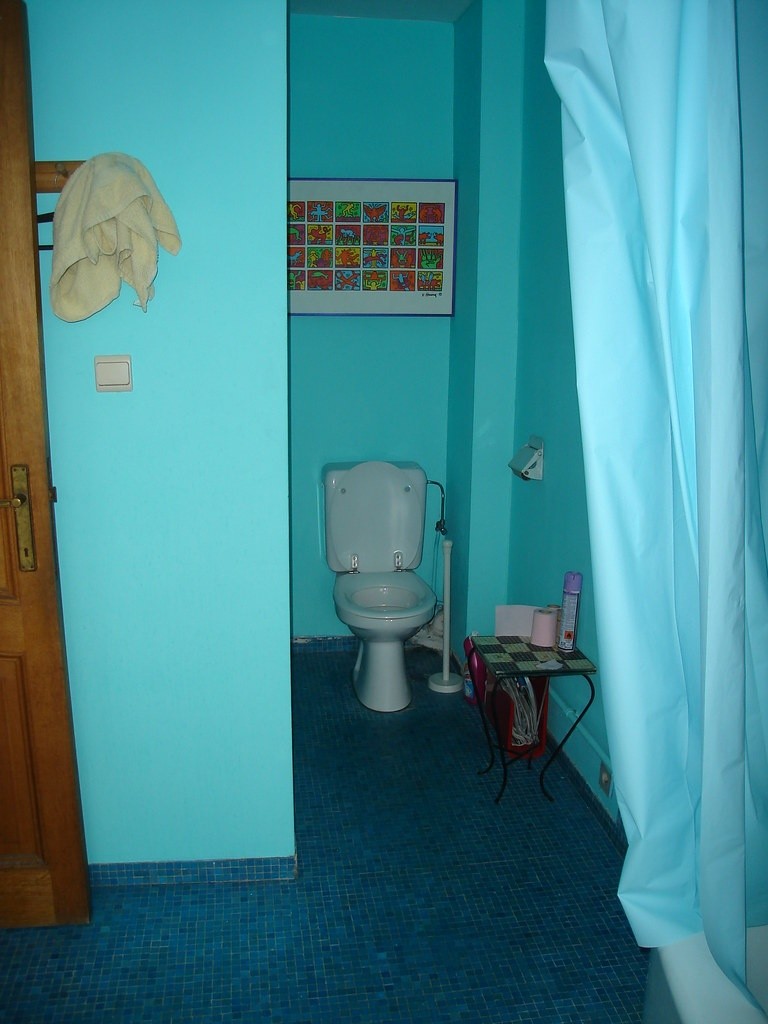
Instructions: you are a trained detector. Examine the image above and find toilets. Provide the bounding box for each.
[320,461,438,713]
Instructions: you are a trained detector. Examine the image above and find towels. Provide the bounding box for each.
[49,152,183,323]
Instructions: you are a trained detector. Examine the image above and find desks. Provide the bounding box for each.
[468,636,596,805]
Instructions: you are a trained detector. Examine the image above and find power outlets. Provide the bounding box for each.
[599,760,614,798]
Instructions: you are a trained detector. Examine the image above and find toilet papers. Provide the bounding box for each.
[495,604,557,648]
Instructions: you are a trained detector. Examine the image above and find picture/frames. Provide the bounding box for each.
[289,177,458,317]
